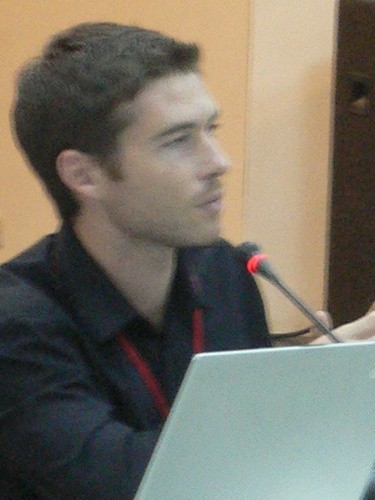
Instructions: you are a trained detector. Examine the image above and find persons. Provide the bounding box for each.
[0,22,375,500]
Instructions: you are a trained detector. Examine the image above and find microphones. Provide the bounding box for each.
[235,242,344,342]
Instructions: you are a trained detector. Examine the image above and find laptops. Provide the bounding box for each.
[133,340,375,500]
